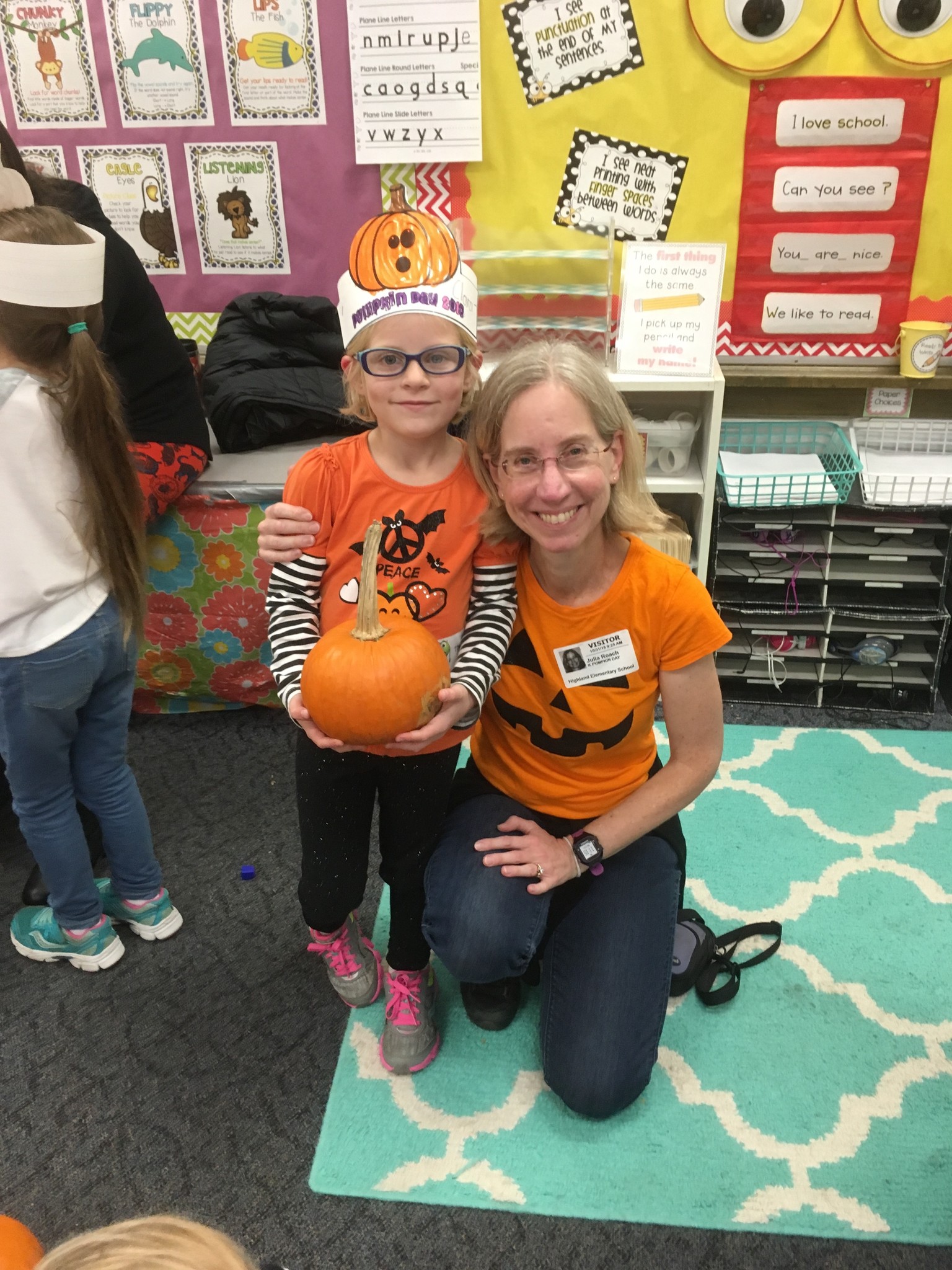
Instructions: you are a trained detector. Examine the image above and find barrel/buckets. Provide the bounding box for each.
[894,320,951,379]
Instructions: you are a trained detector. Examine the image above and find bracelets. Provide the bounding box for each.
[563,836,581,878]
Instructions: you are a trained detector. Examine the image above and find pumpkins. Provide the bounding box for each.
[301,523,451,744]
[0,1213,43,1270]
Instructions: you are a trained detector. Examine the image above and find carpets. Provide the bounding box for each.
[299,713,952,1248]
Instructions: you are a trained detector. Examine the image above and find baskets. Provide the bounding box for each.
[717,420,863,508]
[851,419,952,506]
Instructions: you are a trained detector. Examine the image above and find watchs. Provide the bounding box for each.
[571,829,604,876]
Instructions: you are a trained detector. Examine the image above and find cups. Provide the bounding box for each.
[178,338,200,388]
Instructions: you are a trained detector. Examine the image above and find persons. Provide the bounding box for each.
[256,337,731,1121]
[0,120,214,973]
[263,260,522,1077]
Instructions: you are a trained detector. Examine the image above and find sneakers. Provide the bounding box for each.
[307,910,383,1008]
[92,877,184,941]
[10,905,126,971]
[379,960,439,1073]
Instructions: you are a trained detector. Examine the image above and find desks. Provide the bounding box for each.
[107,418,349,719]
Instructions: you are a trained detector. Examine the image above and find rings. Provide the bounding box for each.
[535,864,543,878]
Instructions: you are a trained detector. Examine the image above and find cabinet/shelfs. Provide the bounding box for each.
[604,316,951,711]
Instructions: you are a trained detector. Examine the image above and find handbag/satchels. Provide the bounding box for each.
[669,908,786,1009]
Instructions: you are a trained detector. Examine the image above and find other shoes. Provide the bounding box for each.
[462,977,521,1031]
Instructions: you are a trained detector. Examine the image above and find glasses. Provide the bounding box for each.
[488,436,618,480]
[351,346,472,377]
